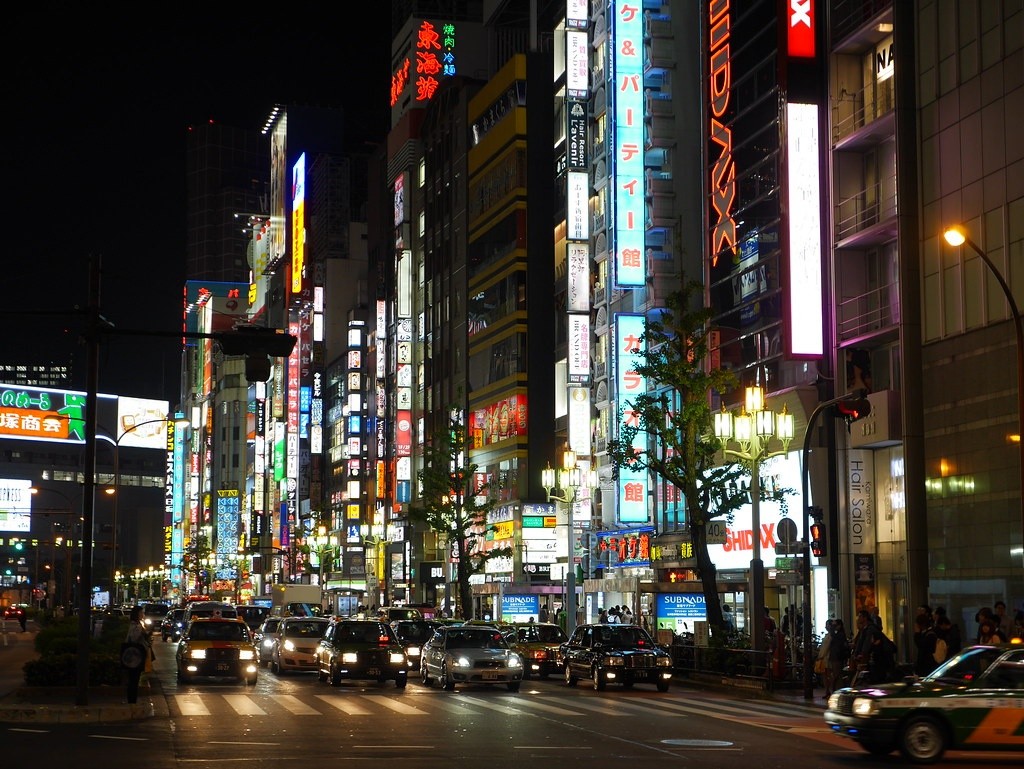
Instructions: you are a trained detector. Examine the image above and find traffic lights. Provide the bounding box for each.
[810,523,828,558]
[838,398,872,421]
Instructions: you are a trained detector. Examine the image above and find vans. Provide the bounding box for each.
[182,600,238,638]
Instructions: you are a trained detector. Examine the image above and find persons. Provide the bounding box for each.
[17,606,27,632]
[914,606,961,677]
[482,604,493,620]
[764,604,802,636]
[540,604,549,622]
[723,605,733,621]
[817,608,898,699]
[600,605,633,624]
[125,606,156,704]
[975,601,1024,645]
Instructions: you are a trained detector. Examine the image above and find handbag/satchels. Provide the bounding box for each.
[121,637,147,668]
[814,659,825,673]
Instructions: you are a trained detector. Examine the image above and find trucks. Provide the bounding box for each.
[271,584,324,618]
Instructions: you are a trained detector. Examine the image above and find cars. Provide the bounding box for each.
[315,620,408,689]
[5,606,22,619]
[382,608,570,680]
[235,605,283,668]
[160,610,185,643]
[390,619,446,671]
[823,636,1024,766]
[560,623,672,693]
[271,616,330,676]
[419,625,524,692]
[176,615,261,686]
[141,603,170,635]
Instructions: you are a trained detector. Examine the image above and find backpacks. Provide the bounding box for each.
[926,631,948,663]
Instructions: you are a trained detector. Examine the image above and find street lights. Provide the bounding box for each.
[28,487,116,605]
[944,224,1024,517]
[41,413,192,606]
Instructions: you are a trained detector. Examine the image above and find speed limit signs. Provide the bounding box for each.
[706,521,727,544]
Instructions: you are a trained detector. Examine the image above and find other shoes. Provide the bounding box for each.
[822,690,832,699]
